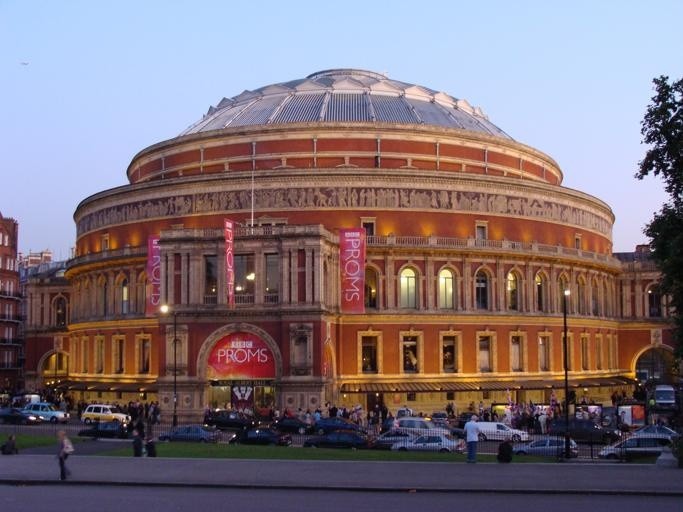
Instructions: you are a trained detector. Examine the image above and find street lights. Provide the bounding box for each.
[562,286,571,459]
[160,305,176,425]
[46,380,60,402]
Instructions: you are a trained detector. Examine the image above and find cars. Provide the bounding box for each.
[0,403,70,425]
[78,404,131,438]
[158,424,222,444]
[272,417,367,451]
[369,430,465,454]
[228,428,292,446]
[511,436,579,459]
[597,425,680,459]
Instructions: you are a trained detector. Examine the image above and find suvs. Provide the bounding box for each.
[391,416,463,440]
[552,420,620,444]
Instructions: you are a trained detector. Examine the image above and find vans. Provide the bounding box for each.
[203,408,257,432]
[655,384,676,412]
[470,421,527,443]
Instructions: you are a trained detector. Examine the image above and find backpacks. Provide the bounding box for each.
[59,438,74,456]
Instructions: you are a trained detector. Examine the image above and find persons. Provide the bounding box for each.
[144,437,157,457]
[239,412,245,420]
[131,430,142,457]
[203,406,218,428]
[446,393,562,432]
[496,437,513,463]
[464,415,480,463]
[2,435,18,455]
[367,403,409,429]
[0,386,160,437]
[56,431,70,481]
[259,404,361,426]
[562,386,648,431]
